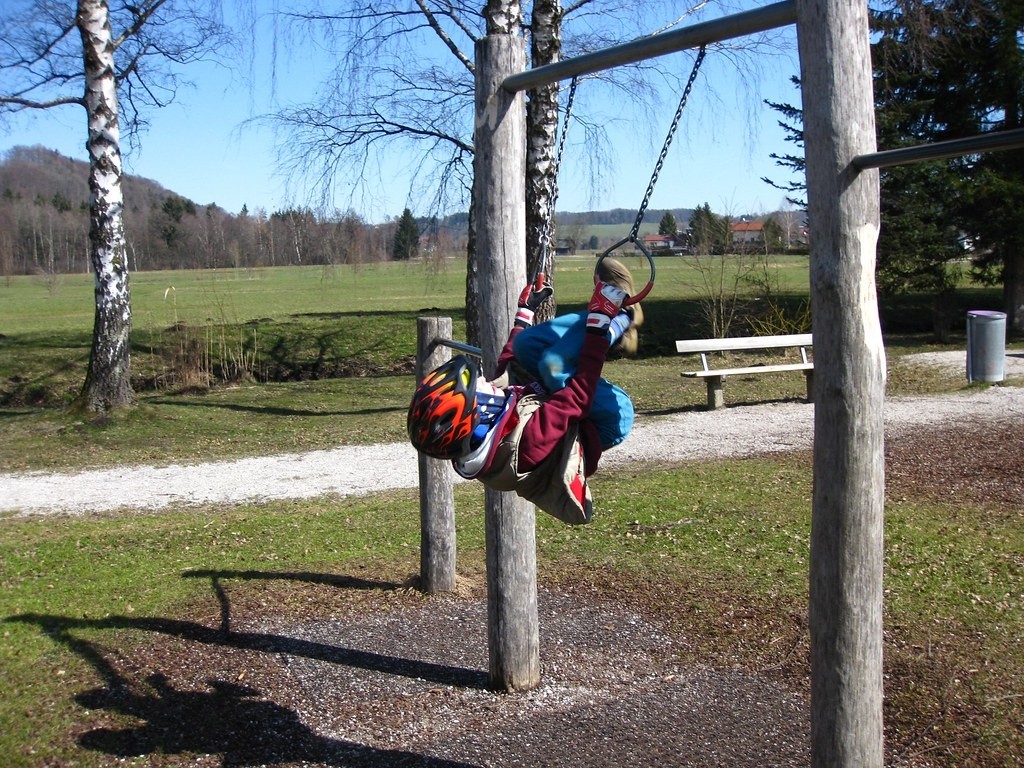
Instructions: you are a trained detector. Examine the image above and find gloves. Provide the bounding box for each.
[585,281,630,336]
[512,279,555,326]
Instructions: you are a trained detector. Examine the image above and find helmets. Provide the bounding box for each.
[405,354,479,459]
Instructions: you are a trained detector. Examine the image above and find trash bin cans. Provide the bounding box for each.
[963,309,1007,388]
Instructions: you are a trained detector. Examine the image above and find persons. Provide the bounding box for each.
[405,256,644,526]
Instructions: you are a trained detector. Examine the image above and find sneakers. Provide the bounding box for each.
[613,325,638,356]
[596,257,644,328]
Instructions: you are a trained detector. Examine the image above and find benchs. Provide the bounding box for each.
[675,334,817,409]
